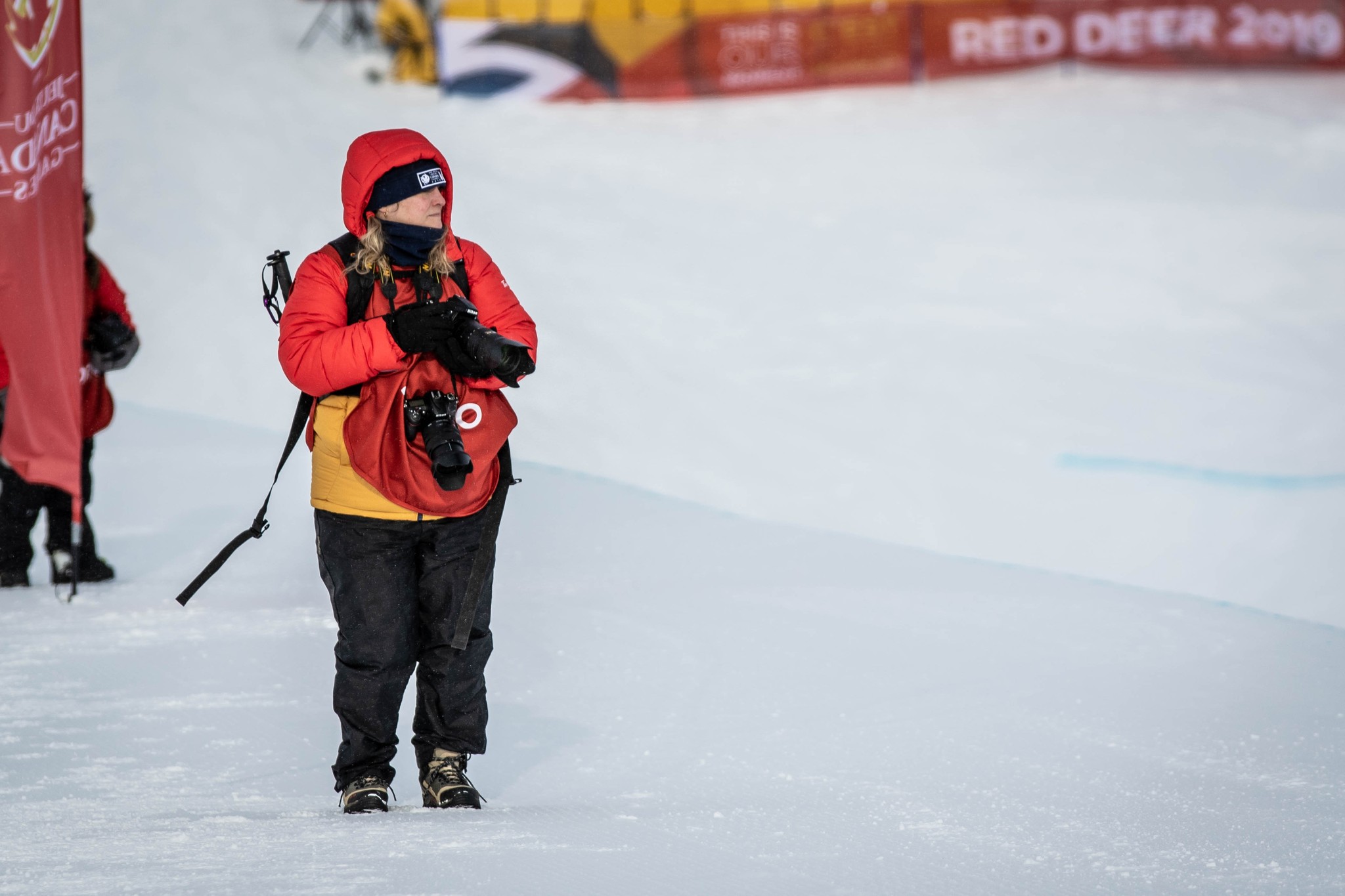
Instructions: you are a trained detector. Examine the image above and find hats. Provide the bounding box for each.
[368,159,446,207]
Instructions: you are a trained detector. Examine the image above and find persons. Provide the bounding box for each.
[0,187,139,590]
[279,129,537,814]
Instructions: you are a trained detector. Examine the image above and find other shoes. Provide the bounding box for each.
[49,556,114,584]
[0,568,29,588]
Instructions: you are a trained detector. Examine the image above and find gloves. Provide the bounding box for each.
[439,344,491,382]
[386,297,453,354]
[88,310,130,354]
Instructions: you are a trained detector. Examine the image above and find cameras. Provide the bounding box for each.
[399,391,474,491]
[435,295,536,388]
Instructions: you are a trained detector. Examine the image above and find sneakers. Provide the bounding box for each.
[339,773,396,814]
[418,747,487,809]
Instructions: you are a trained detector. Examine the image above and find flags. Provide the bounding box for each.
[0,0,84,494]
